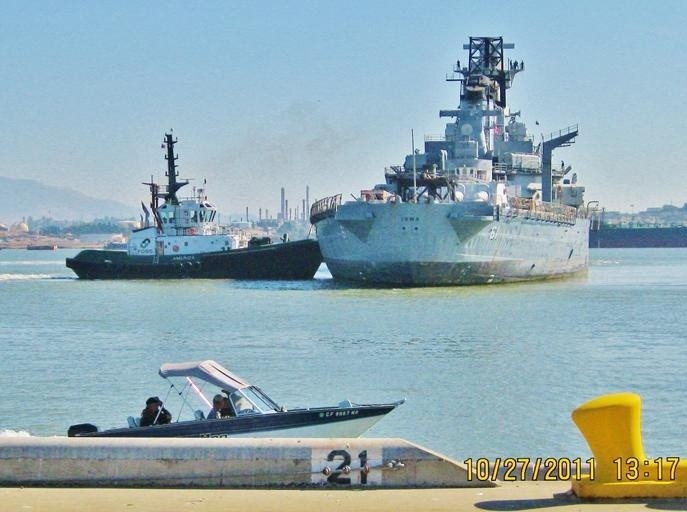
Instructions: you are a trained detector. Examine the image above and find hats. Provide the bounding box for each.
[213,394,226,403]
[146,397,159,404]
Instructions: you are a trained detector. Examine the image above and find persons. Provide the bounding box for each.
[222,391,242,417]
[206,395,226,420]
[138,397,161,427]
[154,395,172,423]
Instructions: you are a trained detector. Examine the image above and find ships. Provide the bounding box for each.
[311,36,600,286]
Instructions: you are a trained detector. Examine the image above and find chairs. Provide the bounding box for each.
[194,410,206,421]
[127,416,140,428]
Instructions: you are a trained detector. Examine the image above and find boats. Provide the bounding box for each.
[67,359,405,439]
[65,133,321,279]
